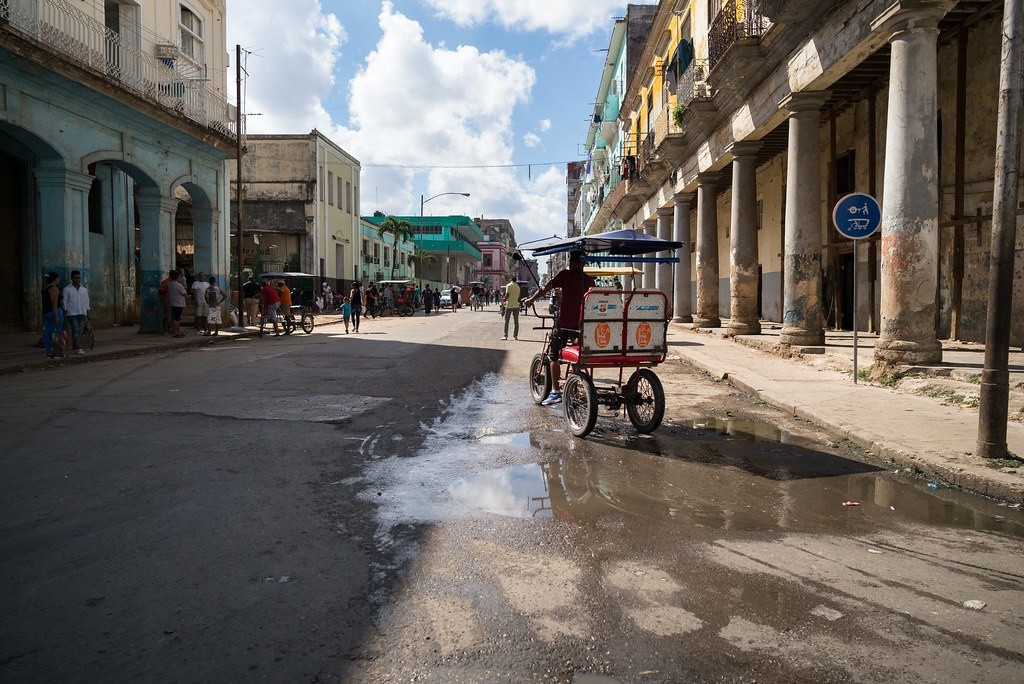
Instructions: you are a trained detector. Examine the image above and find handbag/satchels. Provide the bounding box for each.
[362,304,367,314]
[499,302,506,317]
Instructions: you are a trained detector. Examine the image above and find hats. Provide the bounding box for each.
[570,249,590,265]
[277,280,285,285]
[323,281,327,286]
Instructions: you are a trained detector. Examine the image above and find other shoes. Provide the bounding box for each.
[172,333,184,338]
[77,349,85,355]
[46,354,62,359]
[179,333,186,336]
[194,330,201,335]
[202,330,207,335]
[212,333,218,336]
[501,336,507,340]
[202,333,211,336]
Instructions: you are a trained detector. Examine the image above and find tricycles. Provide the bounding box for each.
[272,305,316,335]
[467,281,485,311]
[512,225,687,440]
[499,281,530,317]
[372,279,416,318]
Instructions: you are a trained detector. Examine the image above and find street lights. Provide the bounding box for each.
[419,192,471,303]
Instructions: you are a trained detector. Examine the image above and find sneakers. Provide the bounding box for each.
[542,390,562,406]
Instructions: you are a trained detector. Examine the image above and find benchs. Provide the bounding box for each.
[560,289,668,366]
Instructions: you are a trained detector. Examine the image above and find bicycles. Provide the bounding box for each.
[81,308,95,350]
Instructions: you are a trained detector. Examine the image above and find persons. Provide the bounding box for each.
[243,276,261,326]
[433,288,442,314]
[422,283,433,316]
[260,279,297,337]
[500,277,520,340]
[410,284,420,308]
[192,271,227,337]
[450,288,458,313]
[157,269,188,338]
[322,282,333,305]
[525,250,596,407]
[336,297,351,334]
[613,276,622,290]
[379,284,395,317]
[485,287,500,306]
[61,270,91,355]
[349,282,362,333]
[469,284,484,299]
[363,282,378,320]
[42,272,69,359]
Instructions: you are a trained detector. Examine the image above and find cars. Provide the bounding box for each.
[438,288,462,309]
